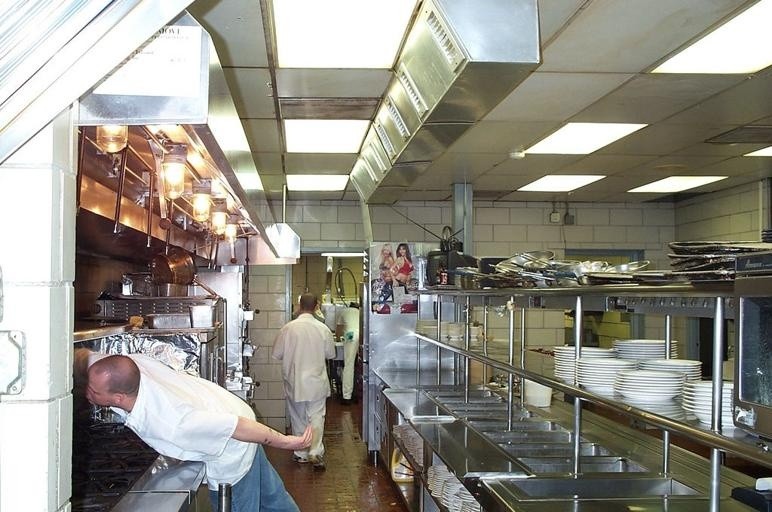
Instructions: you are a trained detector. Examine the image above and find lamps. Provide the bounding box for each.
[92,124,239,245]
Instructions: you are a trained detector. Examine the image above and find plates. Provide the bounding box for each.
[391,422,484,512]
[552,337,735,426]
[413,319,483,342]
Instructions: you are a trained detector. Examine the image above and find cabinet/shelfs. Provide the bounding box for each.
[382,282,772,511]
[373,367,467,468]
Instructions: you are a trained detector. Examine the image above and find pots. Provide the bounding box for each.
[144,246,217,296]
[442,237,772,286]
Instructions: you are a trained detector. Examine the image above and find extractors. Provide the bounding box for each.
[77,171,218,267]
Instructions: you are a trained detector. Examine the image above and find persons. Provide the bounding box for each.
[378,243,395,301]
[391,244,414,295]
[335,301,362,404]
[73,347,315,512]
[270,291,338,471]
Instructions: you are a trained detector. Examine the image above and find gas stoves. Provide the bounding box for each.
[71,421,164,512]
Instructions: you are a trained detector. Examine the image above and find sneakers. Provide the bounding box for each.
[291,454,327,471]
